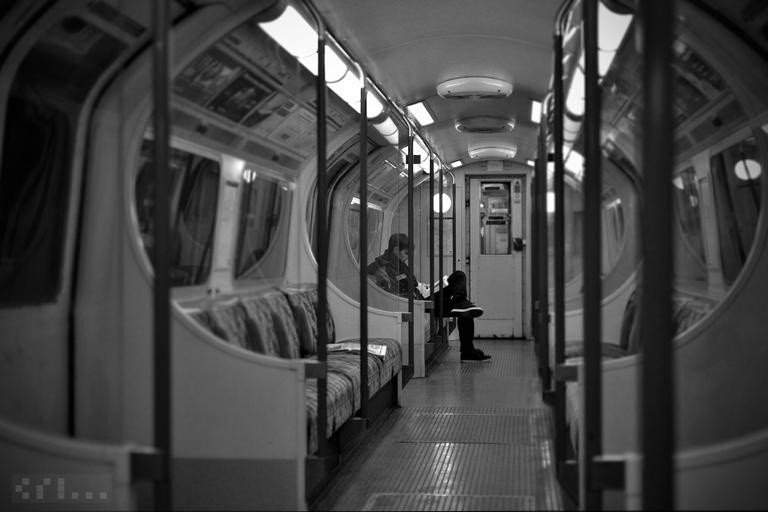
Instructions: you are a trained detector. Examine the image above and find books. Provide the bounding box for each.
[422,275,448,299]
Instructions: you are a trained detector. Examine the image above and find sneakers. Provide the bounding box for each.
[451,303,483,317]
[460,350,491,363]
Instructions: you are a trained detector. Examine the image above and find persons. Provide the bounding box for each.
[365,234,492,363]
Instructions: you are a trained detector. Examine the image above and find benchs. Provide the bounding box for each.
[182,284,402,474]
[564,284,718,364]
